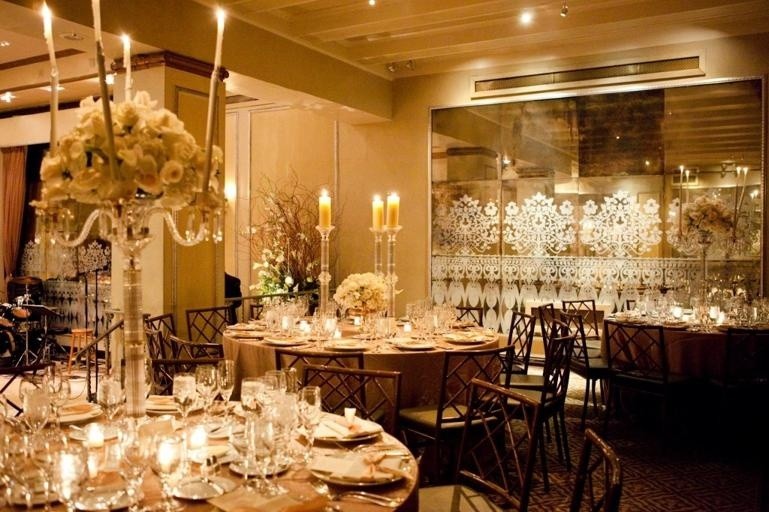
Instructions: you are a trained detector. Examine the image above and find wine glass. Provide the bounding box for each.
[635,295,769,328]
[262,299,458,349]
[0,360,322,512]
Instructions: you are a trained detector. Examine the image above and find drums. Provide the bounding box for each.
[14,321,41,333]
[0,330,23,357]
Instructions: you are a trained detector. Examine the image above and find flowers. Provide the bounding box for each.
[680,196,734,242]
[28,91,229,224]
[333,273,389,314]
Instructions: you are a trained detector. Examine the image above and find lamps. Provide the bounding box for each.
[736,166,749,223]
[560,0,568,17]
[682,168,691,203]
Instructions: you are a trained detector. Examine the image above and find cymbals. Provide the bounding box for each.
[0,316,15,327]
[14,276,41,285]
[13,308,30,318]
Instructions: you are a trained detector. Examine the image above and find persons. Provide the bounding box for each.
[224,271,243,325]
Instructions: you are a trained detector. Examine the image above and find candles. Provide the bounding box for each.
[92,0,119,183]
[201,8,228,194]
[40,0,59,158]
[120,31,132,102]
[733,167,748,236]
[318,188,331,228]
[386,192,400,227]
[677,164,691,241]
[371,194,384,230]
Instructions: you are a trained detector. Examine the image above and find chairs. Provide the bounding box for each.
[7,276,45,370]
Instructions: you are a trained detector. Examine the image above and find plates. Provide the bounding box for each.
[227,320,494,352]
[13,401,411,511]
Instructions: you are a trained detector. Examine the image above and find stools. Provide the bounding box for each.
[67,328,94,375]
